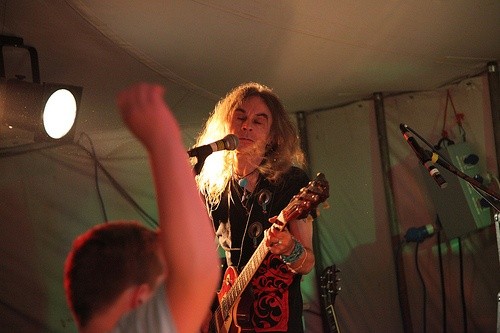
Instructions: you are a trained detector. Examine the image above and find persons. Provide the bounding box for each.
[187,82,315,333]
[65,82,221,333]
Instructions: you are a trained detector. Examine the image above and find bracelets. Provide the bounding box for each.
[279,240,307,275]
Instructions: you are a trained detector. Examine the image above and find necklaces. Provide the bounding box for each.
[234,158,267,187]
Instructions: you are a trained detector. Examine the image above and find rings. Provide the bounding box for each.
[277,240,282,245]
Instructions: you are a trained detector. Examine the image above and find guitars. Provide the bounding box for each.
[319,263,341,333]
[212,170,331,333]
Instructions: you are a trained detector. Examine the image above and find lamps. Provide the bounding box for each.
[0,34,83,143]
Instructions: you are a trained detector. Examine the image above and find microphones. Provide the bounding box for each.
[403,223,435,241]
[188,134,239,157]
[399,124,448,190]
